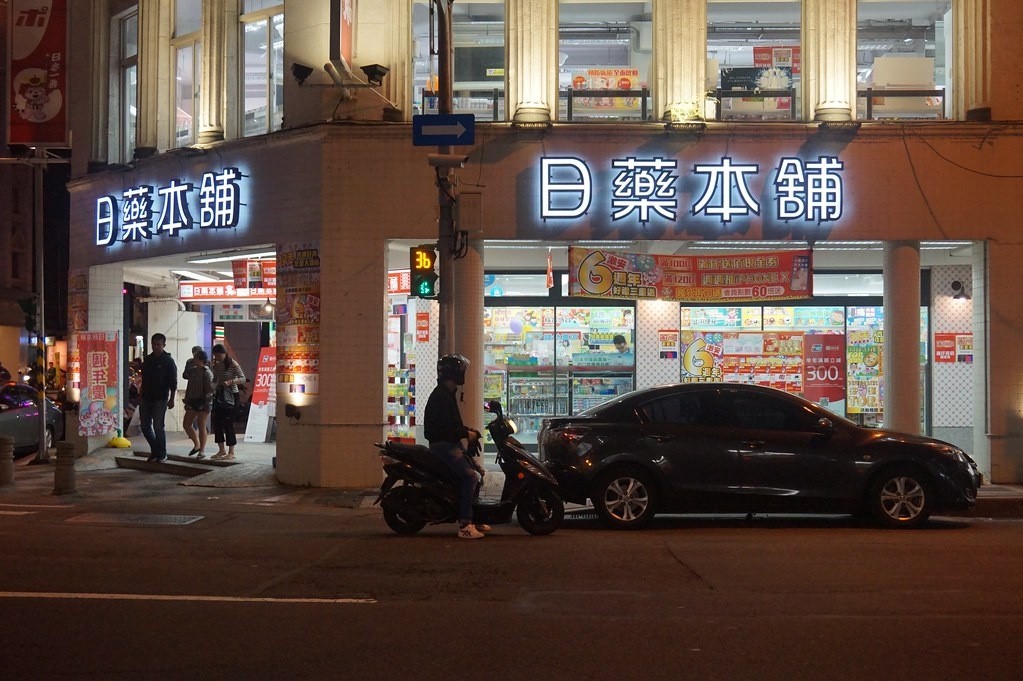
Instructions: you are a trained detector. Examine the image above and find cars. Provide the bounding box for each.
[535,381,984,530]
[0,382,64,450]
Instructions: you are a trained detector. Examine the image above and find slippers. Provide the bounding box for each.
[189,448,200,455]
[211,455,223,460]
[223,454,235,460]
[196,454,206,459]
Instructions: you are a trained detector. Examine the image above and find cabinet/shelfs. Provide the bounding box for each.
[506,367,635,431]
[484,368,502,402]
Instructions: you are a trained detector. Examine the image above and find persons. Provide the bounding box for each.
[0,362,11,381]
[182,346,214,459]
[46,362,56,389]
[423,355,492,539]
[210,344,246,459]
[613,334,633,399]
[139,333,178,462]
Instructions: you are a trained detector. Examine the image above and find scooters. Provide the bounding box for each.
[373,400,566,535]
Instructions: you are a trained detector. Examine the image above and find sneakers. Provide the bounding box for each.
[475,523,491,533]
[458,523,484,538]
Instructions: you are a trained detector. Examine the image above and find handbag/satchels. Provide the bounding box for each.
[213,382,234,409]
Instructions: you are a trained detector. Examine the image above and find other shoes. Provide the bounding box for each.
[157,454,167,463]
[148,455,157,462]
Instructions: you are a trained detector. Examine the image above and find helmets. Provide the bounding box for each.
[437,353,469,385]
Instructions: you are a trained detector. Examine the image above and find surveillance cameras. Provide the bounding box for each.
[951,281,962,291]
[428,154,469,169]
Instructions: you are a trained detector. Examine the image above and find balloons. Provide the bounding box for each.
[522,325,533,341]
[510,319,523,334]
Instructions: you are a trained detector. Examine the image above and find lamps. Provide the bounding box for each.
[290,62,312,85]
[262,298,276,313]
[360,64,390,86]
[952,280,971,301]
[7,142,31,159]
[283,405,301,421]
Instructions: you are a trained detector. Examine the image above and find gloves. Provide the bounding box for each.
[467,427,482,458]
